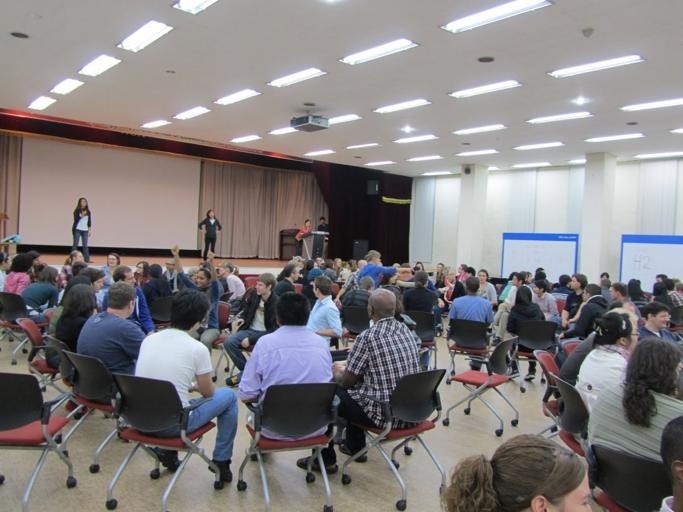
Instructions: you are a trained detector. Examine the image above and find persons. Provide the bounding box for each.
[294,220,312,257]
[1,246,683,511]
[318,217,329,259]
[198,210,222,262]
[72,197,92,263]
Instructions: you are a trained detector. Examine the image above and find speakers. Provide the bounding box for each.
[353,240,368,260]
[366,178,381,196]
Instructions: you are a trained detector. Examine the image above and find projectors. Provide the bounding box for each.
[290,116,330,133]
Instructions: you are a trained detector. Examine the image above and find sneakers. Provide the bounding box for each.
[226,372,242,386]
[469,362,481,372]
[154,447,181,471]
[525,372,535,381]
[491,336,501,346]
[508,370,520,379]
[208,460,232,482]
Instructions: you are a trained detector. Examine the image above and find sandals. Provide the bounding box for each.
[339,444,367,462]
[297,457,338,473]
